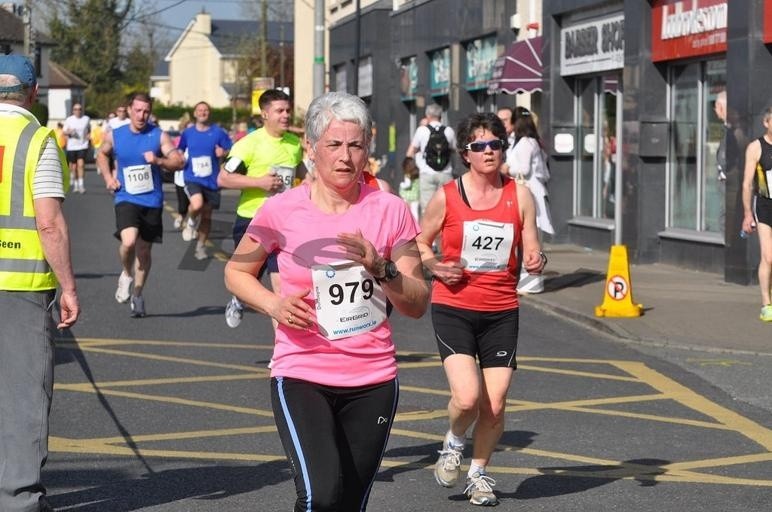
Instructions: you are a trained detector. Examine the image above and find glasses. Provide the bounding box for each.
[465,140,504,152]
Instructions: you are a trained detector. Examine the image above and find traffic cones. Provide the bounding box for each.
[594,245,643,318]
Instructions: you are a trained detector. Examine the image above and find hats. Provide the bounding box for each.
[0,53,35,92]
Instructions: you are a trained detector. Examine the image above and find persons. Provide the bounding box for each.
[0,56,82,512]
[414,110,549,508]
[357,104,557,294]
[53,89,303,328]
[739,105,771,321]
[711,91,729,244]
[579,105,635,225]
[223,89,430,512]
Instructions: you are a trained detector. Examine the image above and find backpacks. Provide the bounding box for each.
[424,124,451,171]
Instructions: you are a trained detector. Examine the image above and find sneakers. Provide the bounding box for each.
[435,431,464,487]
[114,269,133,303]
[195,245,207,261]
[173,216,196,240]
[67,173,86,194]
[759,305,772,321]
[131,295,147,319]
[465,474,497,505]
[224,296,246,329]
